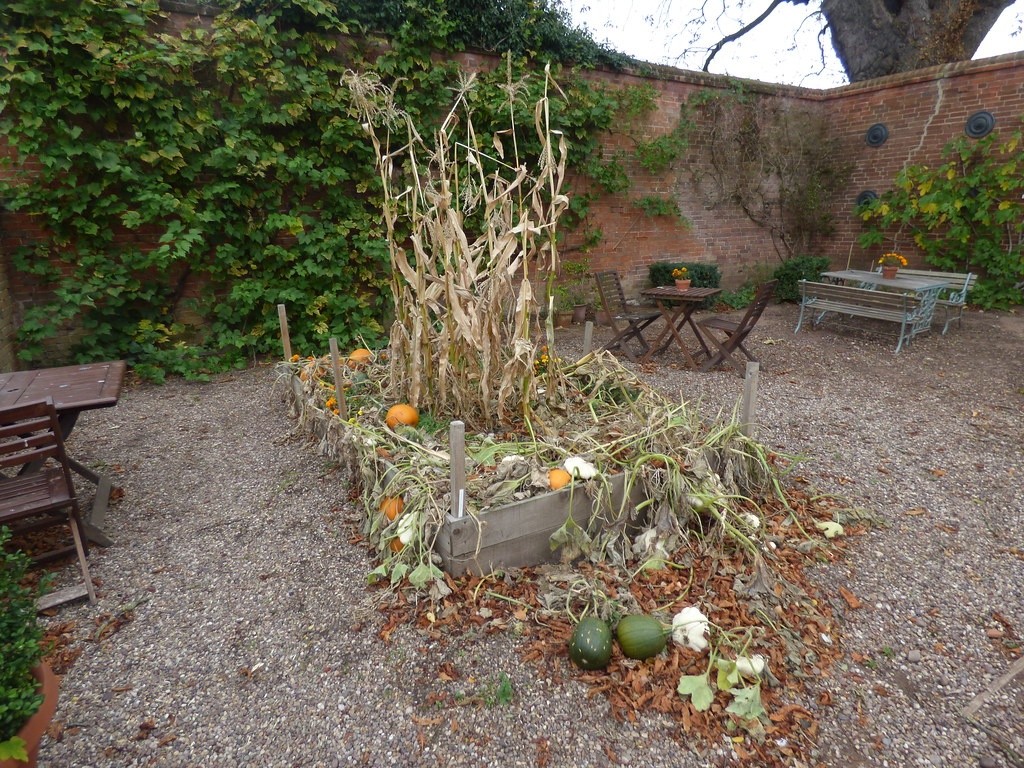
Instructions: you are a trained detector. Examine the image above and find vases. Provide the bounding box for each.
[882,265,898,279]
[675,279,691,292]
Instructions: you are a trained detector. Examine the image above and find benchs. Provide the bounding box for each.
[795,265,978,354]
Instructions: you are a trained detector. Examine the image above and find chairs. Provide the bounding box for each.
[696,279,778,378]
[594,270,662,363]
[0,397,97,615]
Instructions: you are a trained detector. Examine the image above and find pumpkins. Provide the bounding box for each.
[569,616,613,670]
[295,348,776,562]
[616,614,673,659]
[672,607,710,651]
[731,653,765,676]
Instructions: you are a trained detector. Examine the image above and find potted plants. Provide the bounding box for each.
[0,553,59,768]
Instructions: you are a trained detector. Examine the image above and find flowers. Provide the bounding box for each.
[878,253,908,266]
[671,267,687,280]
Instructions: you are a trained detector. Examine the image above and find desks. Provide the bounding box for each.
[0,360,126,546]
[638,286,722,371]
[816,270,948,338]
